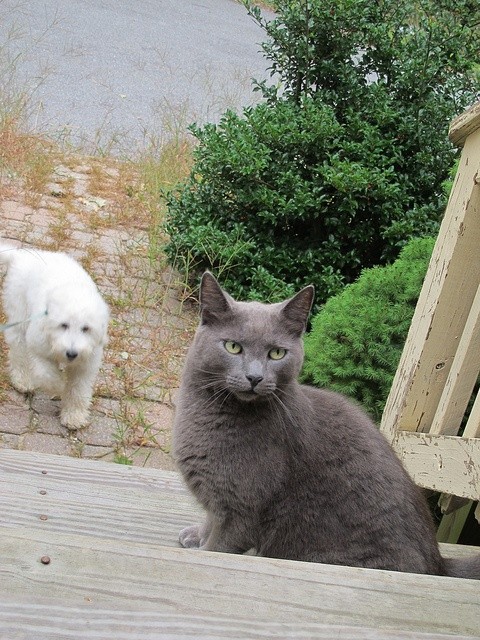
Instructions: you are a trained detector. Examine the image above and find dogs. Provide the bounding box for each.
[0,243,111,432]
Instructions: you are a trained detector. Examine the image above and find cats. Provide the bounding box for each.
[167,269,480,585]
